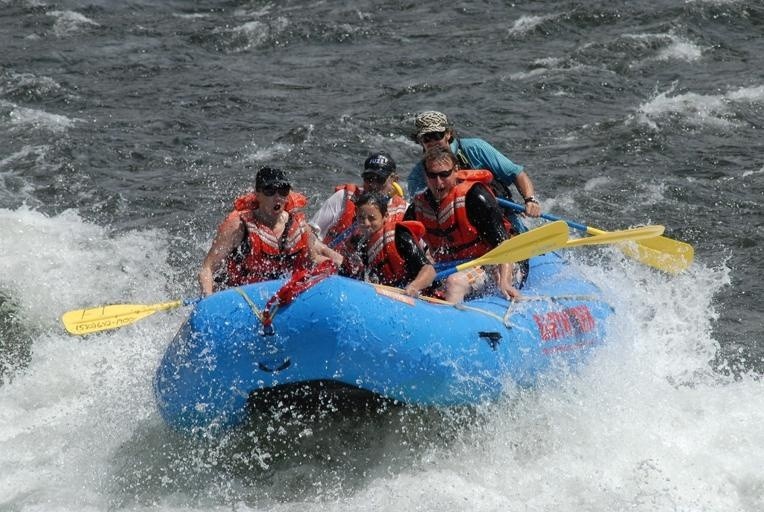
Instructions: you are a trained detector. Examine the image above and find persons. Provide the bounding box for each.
[335,191,437,299]
[402,148,522,307]
[198,165,317,295]
[310,149,409,255]
[409,109,539,236]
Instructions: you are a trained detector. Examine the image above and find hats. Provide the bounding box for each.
[256,167,289,188]
[361,152,396,177]
[417,111,448,138]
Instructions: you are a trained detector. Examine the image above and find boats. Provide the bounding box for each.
[148,237,617,470]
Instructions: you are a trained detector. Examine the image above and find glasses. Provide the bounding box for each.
[256,186,289,196]
[421,129,447,142]
[423,161,455,178]
[364,173,391,184]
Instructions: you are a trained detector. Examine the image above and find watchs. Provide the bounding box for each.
[525,196,538,204]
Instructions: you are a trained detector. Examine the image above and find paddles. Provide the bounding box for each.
[497,197,694,273]
[436,220,569,279]
[440,224,665,269]
[62,295,201,335]
